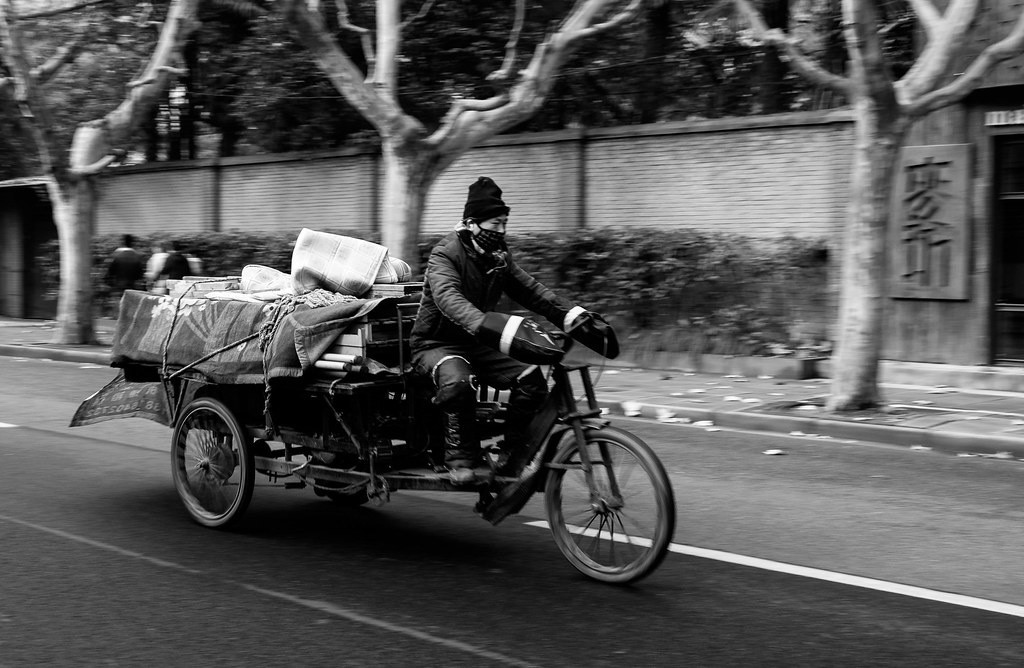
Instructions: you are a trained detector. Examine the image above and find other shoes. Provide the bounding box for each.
[450,465,474,486]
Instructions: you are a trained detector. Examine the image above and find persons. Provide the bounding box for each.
[145,240,203,281]
[106,234,143,290]
[410,177,556,486]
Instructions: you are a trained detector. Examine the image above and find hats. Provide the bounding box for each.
[462,176,510,225]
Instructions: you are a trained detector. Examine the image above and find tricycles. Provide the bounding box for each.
[104,274,678,586]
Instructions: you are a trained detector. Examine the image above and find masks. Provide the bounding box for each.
[470,220,504,254]
